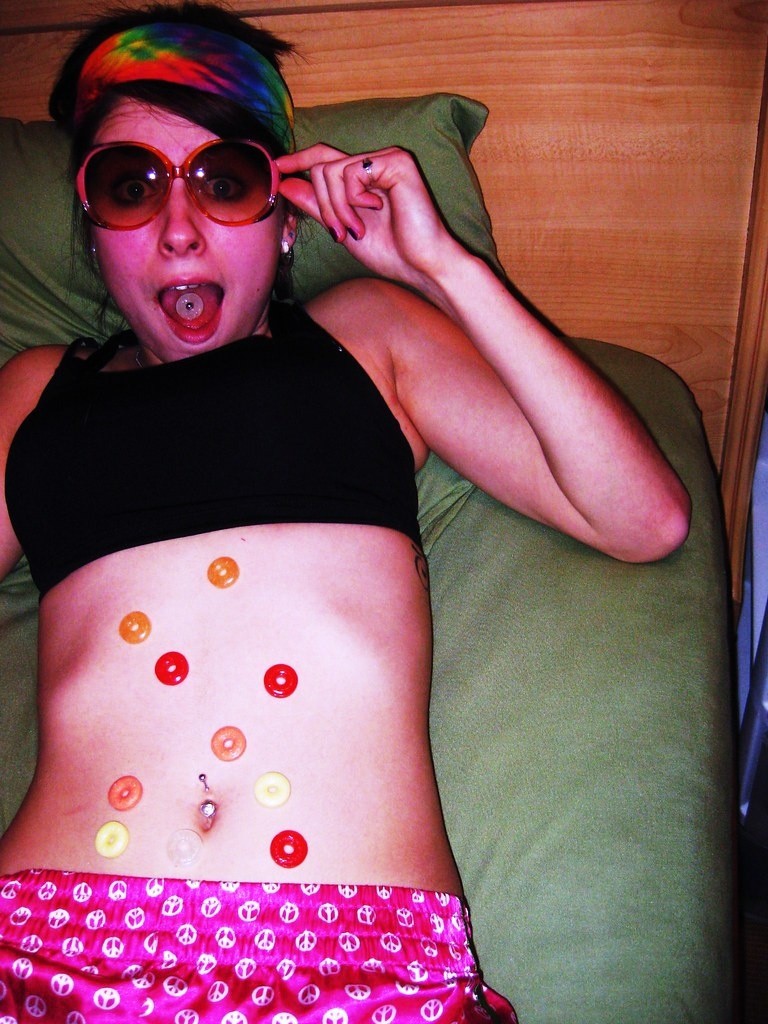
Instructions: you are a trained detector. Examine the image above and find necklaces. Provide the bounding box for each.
[135,347,144,368]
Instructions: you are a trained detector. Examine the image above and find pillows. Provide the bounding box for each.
[0,92,505,572]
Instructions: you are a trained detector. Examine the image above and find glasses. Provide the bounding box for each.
[76,138,281,231]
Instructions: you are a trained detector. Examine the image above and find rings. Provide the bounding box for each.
[361,158,375,186]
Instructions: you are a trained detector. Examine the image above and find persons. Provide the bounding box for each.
[0,0,690,1024]
